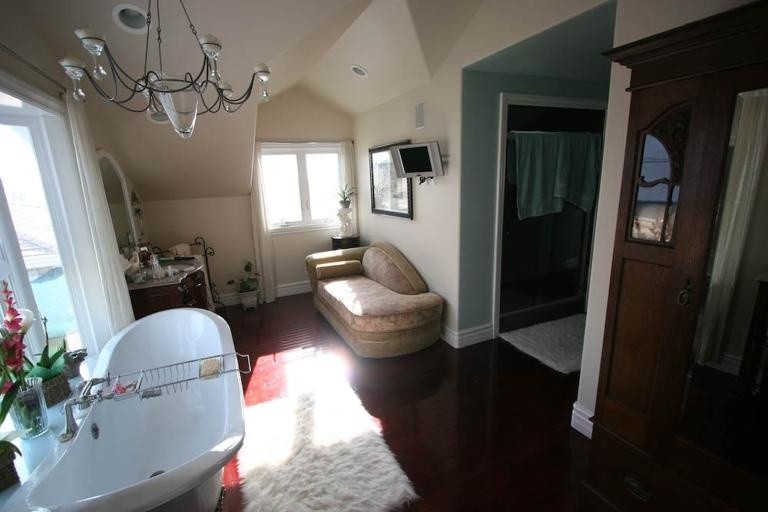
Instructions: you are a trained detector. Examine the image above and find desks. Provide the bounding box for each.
[331,234,361,250]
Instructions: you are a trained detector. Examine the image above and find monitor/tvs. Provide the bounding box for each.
[389,142,444,178]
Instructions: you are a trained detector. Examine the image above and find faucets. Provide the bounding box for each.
[77,375,112,390]
[60,390,103,434]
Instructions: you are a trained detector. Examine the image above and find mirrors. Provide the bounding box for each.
[94,148,142,267]
[367,137,415,222]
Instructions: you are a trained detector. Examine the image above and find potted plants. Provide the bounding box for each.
[337,184,359,208]
[18,336,78,411]
[0,438,24,491]
[226,259,261,312]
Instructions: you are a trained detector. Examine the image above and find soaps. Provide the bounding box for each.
[201,360,220,380]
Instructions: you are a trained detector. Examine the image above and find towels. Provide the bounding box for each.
[505,128,606,222]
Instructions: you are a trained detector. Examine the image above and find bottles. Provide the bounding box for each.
[150,255,165,280]
[168,266,174,281]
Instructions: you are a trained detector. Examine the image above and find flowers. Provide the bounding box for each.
[0,280,38,396]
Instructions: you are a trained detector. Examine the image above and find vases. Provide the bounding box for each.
[0,374,53,444]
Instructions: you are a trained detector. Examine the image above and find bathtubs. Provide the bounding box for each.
[1,305,246,512]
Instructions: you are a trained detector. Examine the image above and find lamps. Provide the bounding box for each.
[54,0,275,143]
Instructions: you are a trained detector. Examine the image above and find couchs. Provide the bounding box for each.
[303,241,446,363]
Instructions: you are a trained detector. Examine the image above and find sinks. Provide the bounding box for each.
[163,263,193,271]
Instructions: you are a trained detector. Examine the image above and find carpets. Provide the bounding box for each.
[495,308,589,377]
[227,373,423,512]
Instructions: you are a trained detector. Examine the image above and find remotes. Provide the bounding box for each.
[174,256,194,260]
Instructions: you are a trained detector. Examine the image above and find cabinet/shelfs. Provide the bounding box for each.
[126,269,214,320]
[735,261,768,395]
